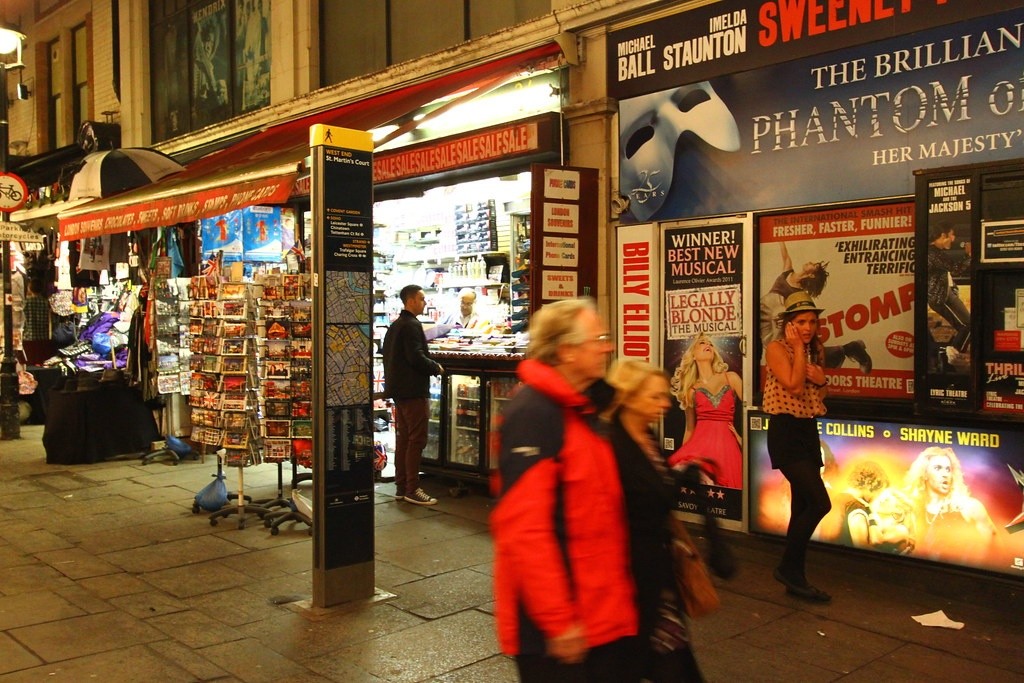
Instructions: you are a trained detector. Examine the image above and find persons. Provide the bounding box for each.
[768,241,873,375]
[155,274,313,469]
[600,359,706,683]
[925,221,971,370]
[23,278,51,367]
[668,332,744,492]
[384,286,443,504]
[816,438,998,557]
[762,295,833,604]
[438,288,480,329]
[190,1,267,119]
[77,288,87,307]
[493,298,639,683]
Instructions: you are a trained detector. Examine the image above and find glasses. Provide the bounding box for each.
[584,333,613,346]
[458,301,474,307]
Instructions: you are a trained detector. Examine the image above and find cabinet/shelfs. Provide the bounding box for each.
[373,198,531,539]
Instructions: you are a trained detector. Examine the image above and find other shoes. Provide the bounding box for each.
[938,347,957,372]
[786,585,832,602]
[771,568,816,597]
[846,339,872,374]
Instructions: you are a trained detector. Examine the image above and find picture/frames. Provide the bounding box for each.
[185,273,313,465]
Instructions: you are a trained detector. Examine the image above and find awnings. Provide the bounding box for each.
[57,48,567,242]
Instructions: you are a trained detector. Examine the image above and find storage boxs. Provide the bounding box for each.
[451,201,473,213]
[200,206,282,263]
[478,242,498,253]
[454,212,476,224]
[476,197,495,209]
[477,208,496,220]
[476,231,500,242]
[475,220,497,232]
[455,224,476,234]
[456,234,477,243]
[457,244,478,253]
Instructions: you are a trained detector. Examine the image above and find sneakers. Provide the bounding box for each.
[394,491,406,499]
[403,488,437,505]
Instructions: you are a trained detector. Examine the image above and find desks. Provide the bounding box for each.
[46,386,153,464]
[26,367,71,425]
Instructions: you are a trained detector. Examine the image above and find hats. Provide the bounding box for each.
[777,292,826,320]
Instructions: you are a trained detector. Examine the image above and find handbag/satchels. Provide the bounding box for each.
[672,515,722,620]
